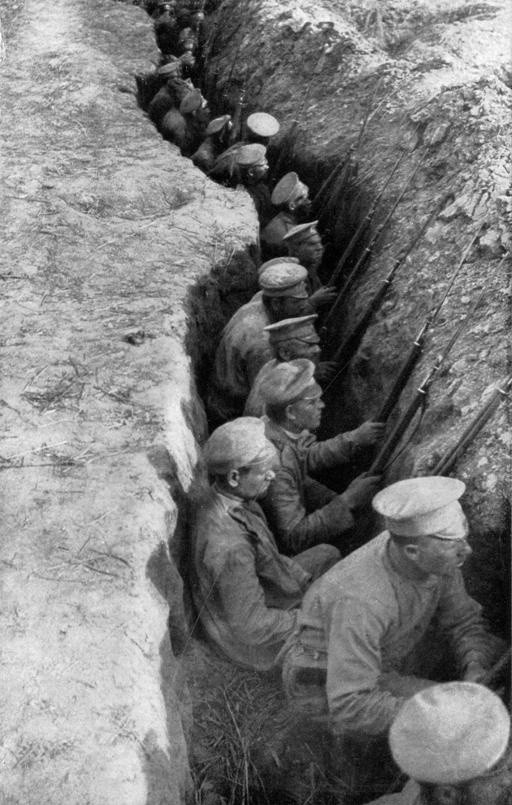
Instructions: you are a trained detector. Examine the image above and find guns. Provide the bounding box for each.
[192,0,317,187]
[308,151,359,232]
[333,202,451,388]
[317,148,431,346]
[376,216,488,422]
[362,251,510,476]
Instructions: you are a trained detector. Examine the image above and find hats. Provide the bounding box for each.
[201,415,277,476]
[180,87,280,166]
[387,680,511,788]
[156,0,205,75]
[371,476,469,540]
[258,171,319,404]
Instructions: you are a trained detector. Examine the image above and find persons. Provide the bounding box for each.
[195,358,512,805]
[200,172,392,468]
[148,1,236,174]
